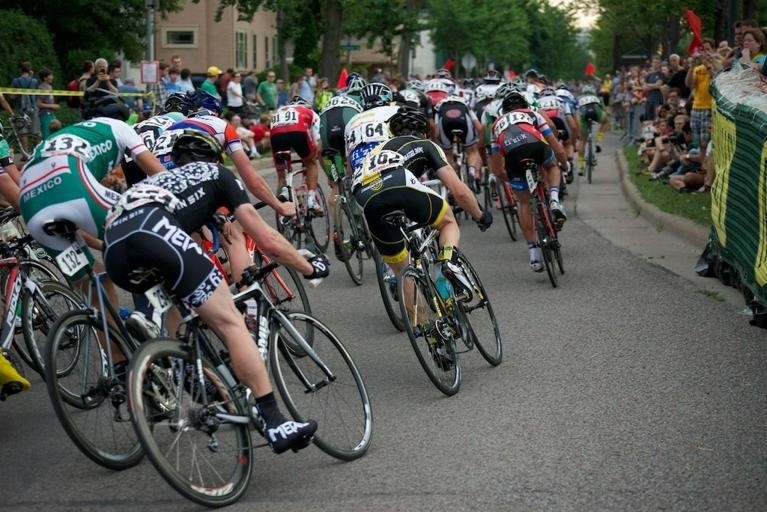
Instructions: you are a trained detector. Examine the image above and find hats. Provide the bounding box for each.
[206,64,224,78]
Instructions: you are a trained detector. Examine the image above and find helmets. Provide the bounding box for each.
[166,91,185,113]
[407,67,598,105]
[171,131,227,166]
[345,72,394,107]
[287,95,311,106]
[181,89,225,117]
[80,88,131,121]
[386,105,432,136]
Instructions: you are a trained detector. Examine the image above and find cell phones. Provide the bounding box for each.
[100,68,105,75]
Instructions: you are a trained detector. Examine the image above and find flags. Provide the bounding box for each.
[685,9,705,54]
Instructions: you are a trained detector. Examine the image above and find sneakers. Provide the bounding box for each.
[487,171,499,204]
[566,161,574,185]
[528,255,545,274]
[124,309,163,347]
[263,419,317,456]
[439,259,474,304]
[467,169,481,195]
[305,189,328,216]
[549,197,569,224]
[418,318,458,374]
[331,227,348,264]
[594,141,603,154]
[0,350,33,393]
[634,138,670,185]
[577,166,586,178]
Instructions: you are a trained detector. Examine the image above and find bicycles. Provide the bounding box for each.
[44,219,270,472]
[124,247,373,508]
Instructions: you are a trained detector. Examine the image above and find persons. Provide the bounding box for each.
[0,22,766,457]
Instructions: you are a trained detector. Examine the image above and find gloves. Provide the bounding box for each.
[471,210,494,232]
[304,254,330,280]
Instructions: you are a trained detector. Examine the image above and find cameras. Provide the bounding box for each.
[695,54,703,59]
